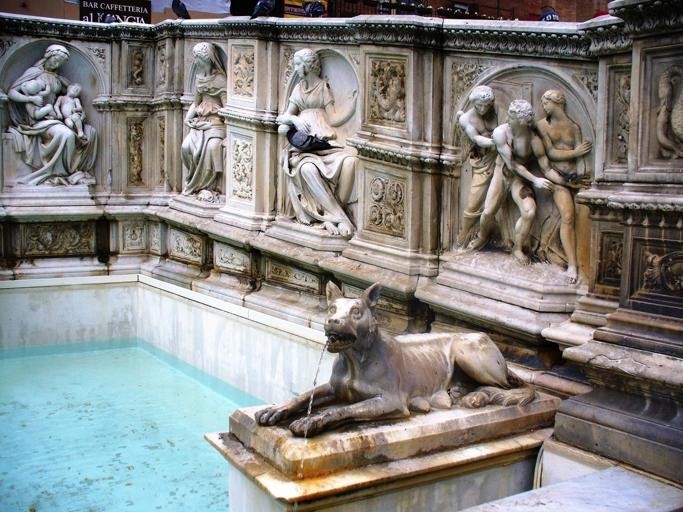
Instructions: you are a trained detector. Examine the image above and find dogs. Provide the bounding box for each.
[252,280,538,439]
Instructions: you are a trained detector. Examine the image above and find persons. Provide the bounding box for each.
[7,45,100,187]
[458,85,592,284]
[181,41,227,204]
[278,49,364,241]
